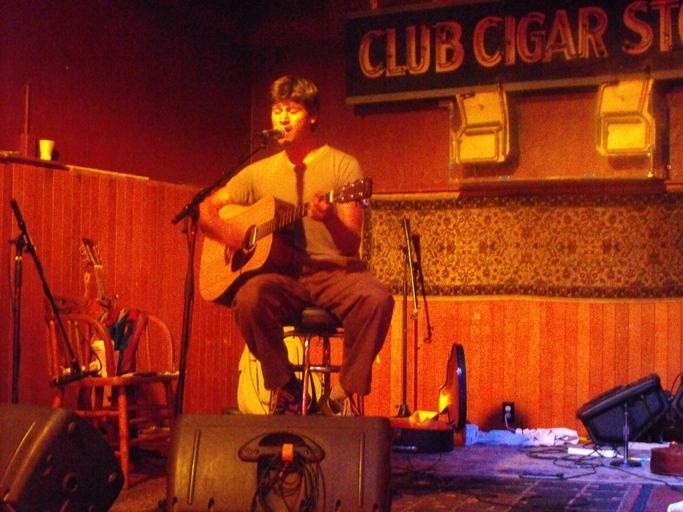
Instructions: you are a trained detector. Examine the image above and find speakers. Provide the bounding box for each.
[166,413,393,511]
[0,402,124,512]
[577,372,669,447]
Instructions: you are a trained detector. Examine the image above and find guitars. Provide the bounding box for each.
[79,238,130,376]
[199,176,372,303]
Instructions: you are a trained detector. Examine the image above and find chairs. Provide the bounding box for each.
[48,309,180,489]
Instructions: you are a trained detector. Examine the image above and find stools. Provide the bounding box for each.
[283,307,369,416]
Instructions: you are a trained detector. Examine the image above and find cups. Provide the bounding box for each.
[39,139,55,161]
[93,339,114,378]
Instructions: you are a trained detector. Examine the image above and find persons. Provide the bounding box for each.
[188,73,395,418]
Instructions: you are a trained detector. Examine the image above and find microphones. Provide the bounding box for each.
[257,128,284,139]
[53,367,97,387]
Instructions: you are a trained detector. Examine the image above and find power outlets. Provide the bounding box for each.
[503,401,515,423]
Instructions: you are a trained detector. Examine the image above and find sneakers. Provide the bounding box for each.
[320,397,340,415]
[271,382,311,415]
